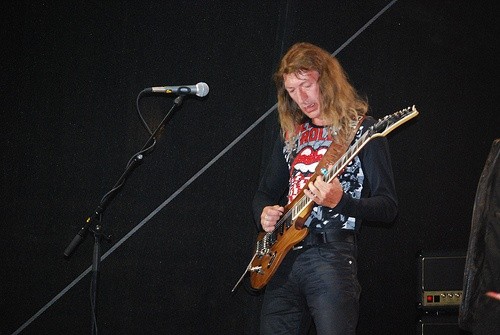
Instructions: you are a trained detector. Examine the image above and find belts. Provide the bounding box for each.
[289,229,359,257]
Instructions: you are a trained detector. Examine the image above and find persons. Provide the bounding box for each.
[251,41,399,334]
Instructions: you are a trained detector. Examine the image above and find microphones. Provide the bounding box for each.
[145,82,210,98]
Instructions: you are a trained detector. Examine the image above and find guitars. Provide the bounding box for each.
[248,105,419,289]
